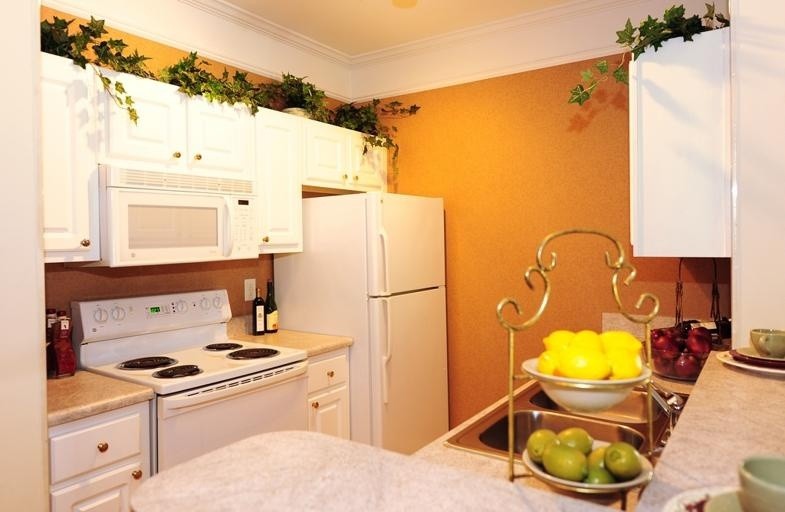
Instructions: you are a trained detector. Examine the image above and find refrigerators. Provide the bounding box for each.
[273,189,451,455]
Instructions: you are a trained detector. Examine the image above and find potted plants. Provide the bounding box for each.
[241,70,329,118]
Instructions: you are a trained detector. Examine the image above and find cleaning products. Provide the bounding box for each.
[53,307,76,378]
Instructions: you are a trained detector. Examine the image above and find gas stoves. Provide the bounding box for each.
[84,336,309,396]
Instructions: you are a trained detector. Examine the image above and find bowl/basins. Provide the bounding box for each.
[522,438,654,495]
[521,357,653,414]
[750,329,785,358]
[641,340,731,381]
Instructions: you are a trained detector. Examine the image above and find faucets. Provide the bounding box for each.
[646,380,685,446]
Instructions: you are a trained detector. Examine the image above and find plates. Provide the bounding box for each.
[664,486,743,511]
[715,346,785,375]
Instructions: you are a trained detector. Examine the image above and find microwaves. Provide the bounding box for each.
[97,165,261,271]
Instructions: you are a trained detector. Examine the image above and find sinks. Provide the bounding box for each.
[515,383,689,426]
[443,408,668,461]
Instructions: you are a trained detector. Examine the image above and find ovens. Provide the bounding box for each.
[157,362,311,472]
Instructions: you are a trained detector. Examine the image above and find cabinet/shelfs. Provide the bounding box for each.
[39,51,102,266]
[623,23,732,260]
[301,117,386,194]
[48,399,151,512]
[97,66,255,180]
[254,103,304,255]
[308,345,350,440]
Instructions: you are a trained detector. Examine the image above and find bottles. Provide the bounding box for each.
[46,309,75,378]
[251,288,265,336]
[265,280,279,332]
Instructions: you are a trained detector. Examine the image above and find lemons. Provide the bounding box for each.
[526,428,642,484]
[536,330,644,379]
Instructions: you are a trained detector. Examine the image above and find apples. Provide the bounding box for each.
[651,327,712,377]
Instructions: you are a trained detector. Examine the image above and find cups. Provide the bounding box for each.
[737,457,785,511]
